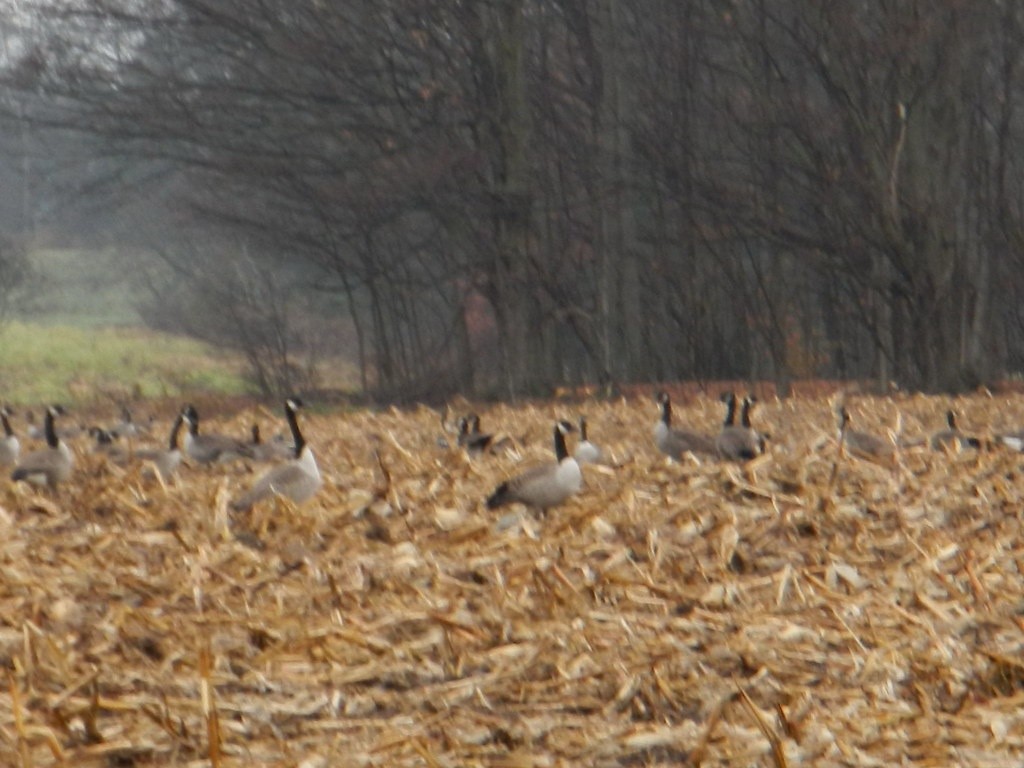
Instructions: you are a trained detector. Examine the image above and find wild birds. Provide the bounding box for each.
[454,412,512,472]
[238,397,324,511]
[0,401,298,505]
[827,404,1024,476]
[574,414,605,468]
[651,390,767,469]
[482,419,583,534]
